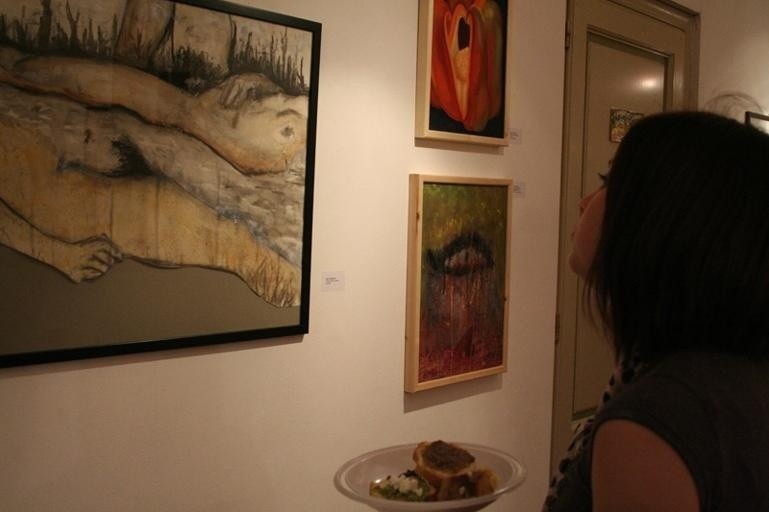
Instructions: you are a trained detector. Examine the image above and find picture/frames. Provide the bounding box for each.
[414,1,511,147]
[404,173,514,395]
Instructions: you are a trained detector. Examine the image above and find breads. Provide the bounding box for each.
[368,438,498,503]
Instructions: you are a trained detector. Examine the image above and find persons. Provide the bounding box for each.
[542,111,769,511]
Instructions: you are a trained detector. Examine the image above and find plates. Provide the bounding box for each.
[332,441,528,511]
